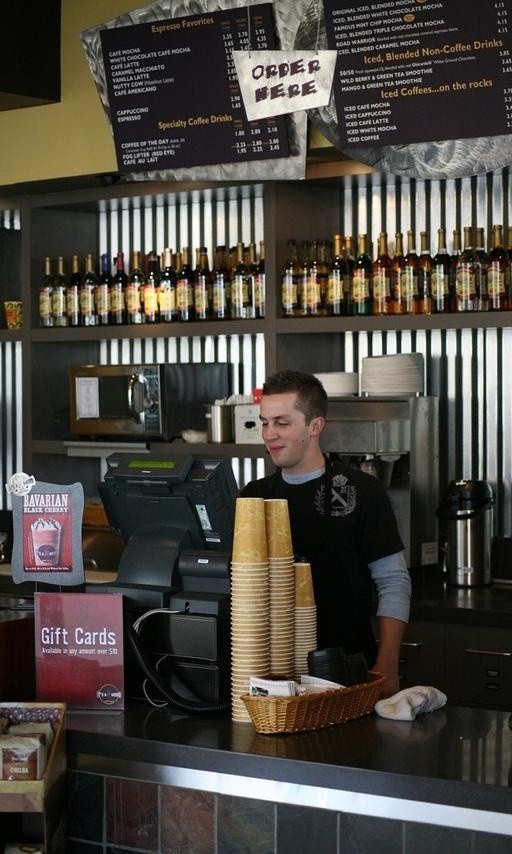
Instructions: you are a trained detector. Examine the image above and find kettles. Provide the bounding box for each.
[437,476,495,591]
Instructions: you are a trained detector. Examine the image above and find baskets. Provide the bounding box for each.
[239,671,384,735]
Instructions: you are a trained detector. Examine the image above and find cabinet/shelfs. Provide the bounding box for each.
[1,179,512,462]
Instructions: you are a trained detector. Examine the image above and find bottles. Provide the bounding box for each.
[391,232,405,315]
[230,242,250,319]
[126,251,144,325]
[351,234,373,316]
[280,237,332,318]
[193,246,210,322]
[38,256,52,328]
[374,232,392,316]
[418,232,432,315]
[487,224,512,311]
[175,248,194,323]
[210,245,231,321]
[451,226,488,312]
[248,240,265,319]
[429,228,452,313]
[327,234,356,317]
[141,250,161,324]
[112,251,128,326]
[66,255,80,327]
[80,254,97,327]
[96,253,113,326]
[52,256,68,329]
[159,247,176,323]
[401,229,420,316]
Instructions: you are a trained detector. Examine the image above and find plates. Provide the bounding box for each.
[313,372,358,397]
[361,353,424,396]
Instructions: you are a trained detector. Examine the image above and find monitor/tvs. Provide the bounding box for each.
[94,449,243,596]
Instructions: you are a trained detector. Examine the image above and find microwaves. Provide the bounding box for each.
[69,363,228,439]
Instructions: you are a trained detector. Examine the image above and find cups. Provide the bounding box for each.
[4,302,22,328]
[30,529,62,568]
[265,499,294,678]
[228,498,270,724]
[294,563,317,681]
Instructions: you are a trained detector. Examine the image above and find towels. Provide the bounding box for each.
[374,684,448,721]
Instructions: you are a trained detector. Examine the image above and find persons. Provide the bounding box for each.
[239,370,412,702]
